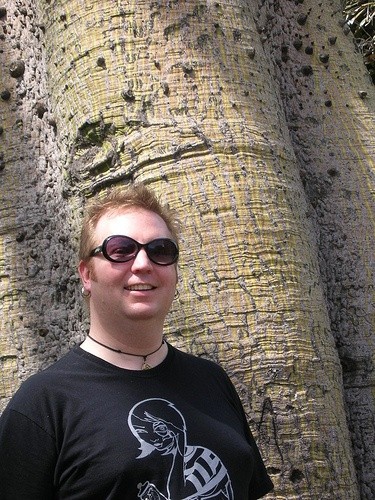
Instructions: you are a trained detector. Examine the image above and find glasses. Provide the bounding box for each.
[89,235,181,266]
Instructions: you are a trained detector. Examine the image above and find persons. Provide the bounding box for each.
[0,182,275,500]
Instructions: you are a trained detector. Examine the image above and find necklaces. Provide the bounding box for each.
[83,335,165,370]
